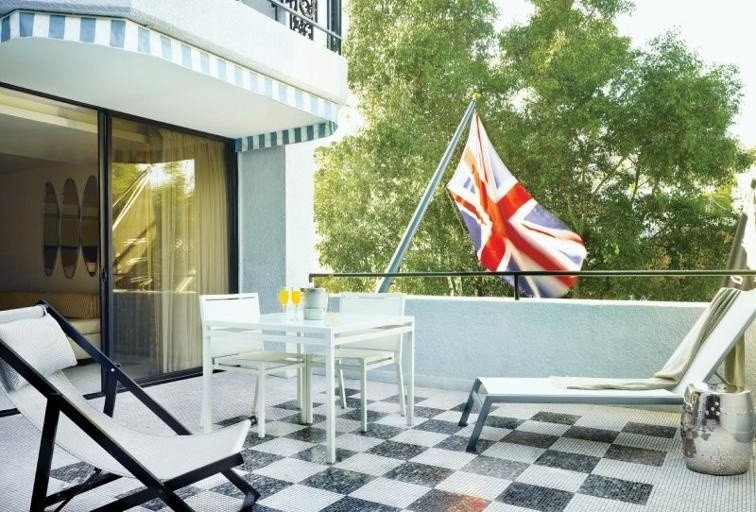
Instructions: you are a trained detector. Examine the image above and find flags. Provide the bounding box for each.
[447,110,588,299]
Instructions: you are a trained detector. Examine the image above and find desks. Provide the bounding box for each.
[203,312,418,463]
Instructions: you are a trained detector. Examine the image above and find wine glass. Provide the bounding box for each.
[291,287,303,320]
[279,287,290,320]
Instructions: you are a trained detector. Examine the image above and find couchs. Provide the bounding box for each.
[2,291,100,361]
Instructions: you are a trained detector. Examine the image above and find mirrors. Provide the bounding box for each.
[40,176,98,278]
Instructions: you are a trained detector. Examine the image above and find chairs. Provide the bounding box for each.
[456,285,754,453]
[200,292,408,435]
[0,300,262,512]
